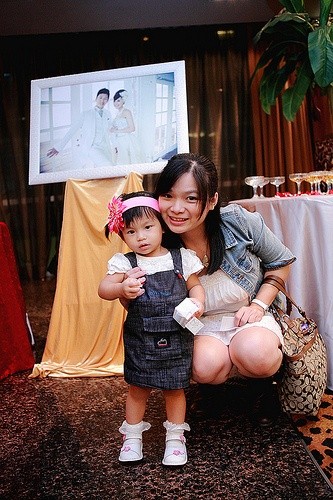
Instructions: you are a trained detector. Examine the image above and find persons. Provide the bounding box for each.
[123,154,326,429]
[97,192,206,466]
[46,87,161,171]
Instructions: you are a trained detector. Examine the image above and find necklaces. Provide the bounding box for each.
[185,238,209,269]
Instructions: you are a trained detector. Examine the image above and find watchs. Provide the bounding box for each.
[251,298,269,315]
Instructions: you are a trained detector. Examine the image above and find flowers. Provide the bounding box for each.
[106,195,127,234]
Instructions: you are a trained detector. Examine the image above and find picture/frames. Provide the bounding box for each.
[29,60,190,185]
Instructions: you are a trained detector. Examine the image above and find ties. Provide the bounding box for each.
[99,110,103,118]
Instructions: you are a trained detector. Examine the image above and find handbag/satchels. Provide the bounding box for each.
[263,275,328,415]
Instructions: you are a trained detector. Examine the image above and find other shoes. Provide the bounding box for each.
[162,431,187,465]
[118,436,143,461]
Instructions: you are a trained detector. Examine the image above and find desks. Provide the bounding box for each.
[228,194,333,391]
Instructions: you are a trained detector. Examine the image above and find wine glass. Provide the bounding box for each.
[269,176,285,197]
[258,178,269,198]
[304,171,333,195]
[245,176,265,199]
[289,173,304,196]
